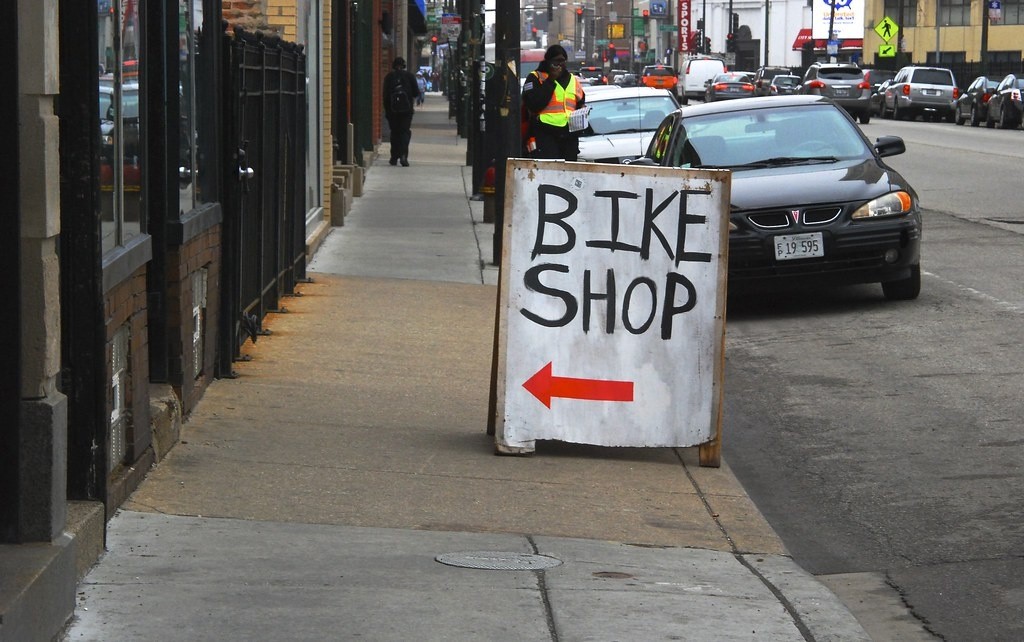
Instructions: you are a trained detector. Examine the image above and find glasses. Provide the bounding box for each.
[551,58,566,63]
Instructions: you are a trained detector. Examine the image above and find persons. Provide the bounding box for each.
[432,69,439,91]
[416,71,427,106]
[422,69,429,79]
[383,57,420,166]
[522,45,585,162]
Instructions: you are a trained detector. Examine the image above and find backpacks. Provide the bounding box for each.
[390,74,412,113]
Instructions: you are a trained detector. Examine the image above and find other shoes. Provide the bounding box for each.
[400,159,409,166]
[389,157,397,165]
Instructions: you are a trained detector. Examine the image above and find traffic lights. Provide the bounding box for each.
[643,10,649,24]
[703,37,712,54]
[695,32,701,45]
[532,27,537,37]
[590,19,594,38]
[726,33,736,52]
[577,9,583,23]
[609,43,616,59]
[431,36,437,42]
[639,42,645,50]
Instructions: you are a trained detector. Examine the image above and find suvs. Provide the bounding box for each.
[792,62,898,124]
[879,66,959,121]
[752,67,791,96]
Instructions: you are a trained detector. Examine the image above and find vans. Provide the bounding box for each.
[676,58,728,105]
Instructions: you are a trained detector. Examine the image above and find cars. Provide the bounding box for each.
[626,96,923,300]
[955,73,1024,129]
[640,64,677,89]
[567,67,641,86]
[871,79,893,117]
[578,79,681,163]
[768,74,802,96]
[704,72,755,102]
